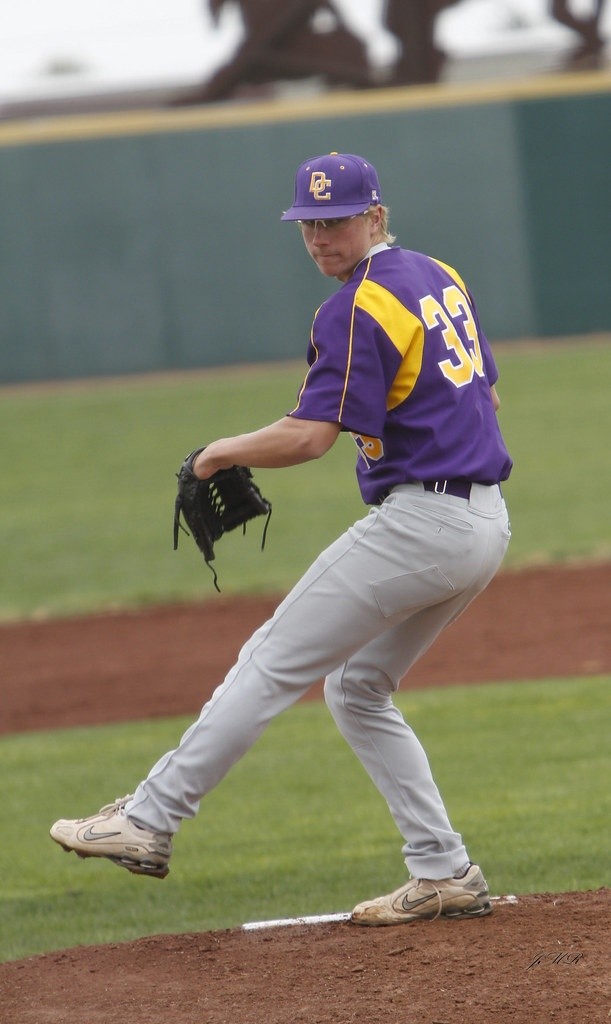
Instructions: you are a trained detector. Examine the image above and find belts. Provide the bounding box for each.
[423,480,503,500]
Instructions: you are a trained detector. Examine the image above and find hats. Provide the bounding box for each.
[281,152,381,221]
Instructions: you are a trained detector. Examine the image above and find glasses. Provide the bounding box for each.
[298,210,368,226]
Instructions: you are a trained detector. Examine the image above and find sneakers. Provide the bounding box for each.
[351,862,492,927]
[49,795,169,879]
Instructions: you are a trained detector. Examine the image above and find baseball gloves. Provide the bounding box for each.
[176,445,267,561]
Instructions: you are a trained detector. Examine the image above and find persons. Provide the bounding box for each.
[49,152,514,925]
